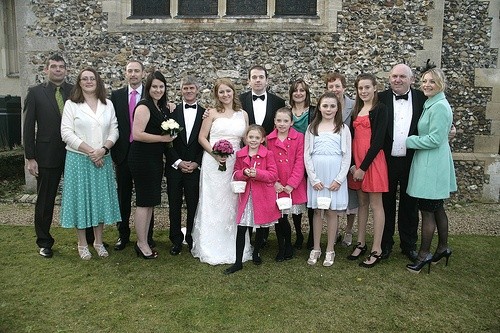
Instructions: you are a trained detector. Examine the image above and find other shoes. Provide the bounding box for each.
[295,235,315,250]
[276,246,292,261]
[331,231,353,246]
[223,257,261,274]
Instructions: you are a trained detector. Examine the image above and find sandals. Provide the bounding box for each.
[77,242,109,258]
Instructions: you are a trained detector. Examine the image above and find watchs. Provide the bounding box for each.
[101,145,110,155]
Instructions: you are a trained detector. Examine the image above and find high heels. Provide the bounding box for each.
[406,253,433,275]
[134,242,158,259]
[430,248,451,266]
[307,250,335,267]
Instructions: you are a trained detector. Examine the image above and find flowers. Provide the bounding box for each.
[161,118,180,139]
[213,139,234,172]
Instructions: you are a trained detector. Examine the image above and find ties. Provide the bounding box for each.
[55,87,65,116]
[129,90,137,143]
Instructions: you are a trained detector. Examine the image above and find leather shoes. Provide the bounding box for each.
[379,247,394,258]
[39,248,52,258]
[170,244,182,254]
[403,249,418,261]
[348,243,383,268]
[115,238,128,249]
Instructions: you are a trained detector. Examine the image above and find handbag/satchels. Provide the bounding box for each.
[230,170,246,194]
[317,186,332,210]
[276,187,292,210]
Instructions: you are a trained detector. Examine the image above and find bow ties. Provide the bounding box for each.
[185,104,197,109]
[252,95,265,101]
[395,94,408,100]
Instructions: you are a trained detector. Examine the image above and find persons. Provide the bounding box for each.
[110,61,157,250]
[201,65,286,251]
[405,59,459,274]
[265,108,308,262]
[288,78,318,250]
[348,74,390,268]
[164,74,211,256]
[190,78,260,268]
[22,54,110,258]
[128,70,178,259]
[377,63,457,264]
[303,93,352,266]
[325,72,358,248]
[57,67,123,260]
[222,124,278,275]
